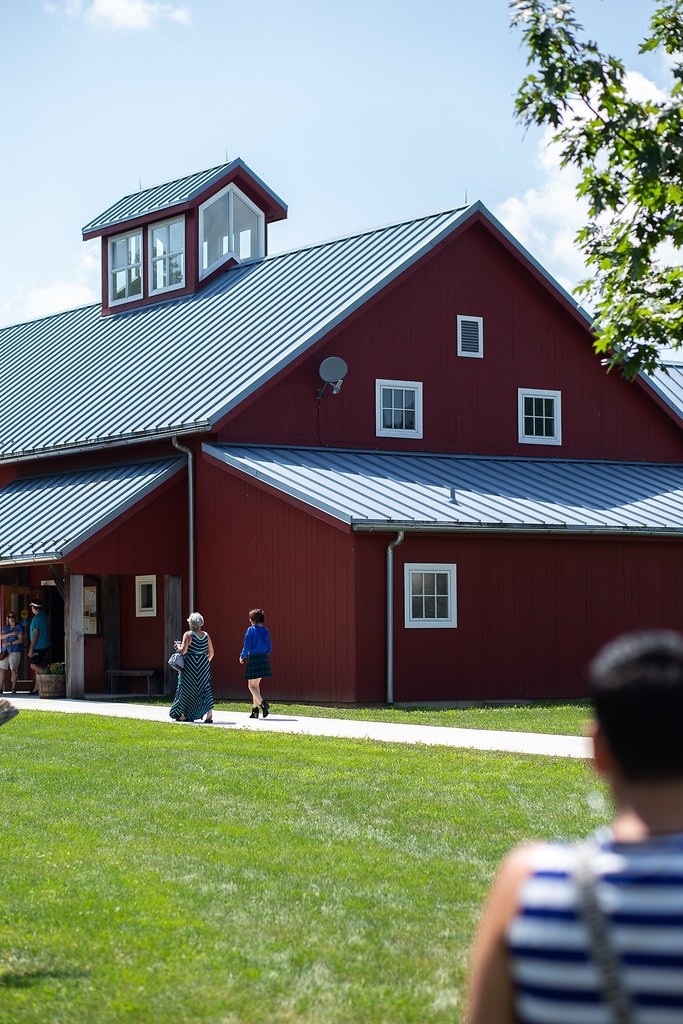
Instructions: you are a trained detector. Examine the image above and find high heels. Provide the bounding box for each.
[260,701,270,719]
[250,706,260,719]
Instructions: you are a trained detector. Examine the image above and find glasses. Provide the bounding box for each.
[7,615,16,619]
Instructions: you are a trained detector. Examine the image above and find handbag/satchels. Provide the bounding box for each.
[167,650,185,671]
[0,645,10,662]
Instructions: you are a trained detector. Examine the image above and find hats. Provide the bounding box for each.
[28,600,44,611]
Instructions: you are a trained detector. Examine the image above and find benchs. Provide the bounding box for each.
[107,668,158,695]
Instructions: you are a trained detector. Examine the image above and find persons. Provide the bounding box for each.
[465,627,683,1024]
[0,610,24,695]
[169,611,215,723]
[28,599,50,695]
[238,608,272,719]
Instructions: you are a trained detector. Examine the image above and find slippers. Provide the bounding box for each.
[204,716,213,723]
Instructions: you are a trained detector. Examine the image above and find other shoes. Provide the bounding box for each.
[0,689,3,696]
[11,688,16,695]
[29,688,39,695]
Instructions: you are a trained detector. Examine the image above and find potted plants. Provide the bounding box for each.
[36,662,68,699]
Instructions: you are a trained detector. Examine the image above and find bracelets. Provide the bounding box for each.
[10,641,13,646]
